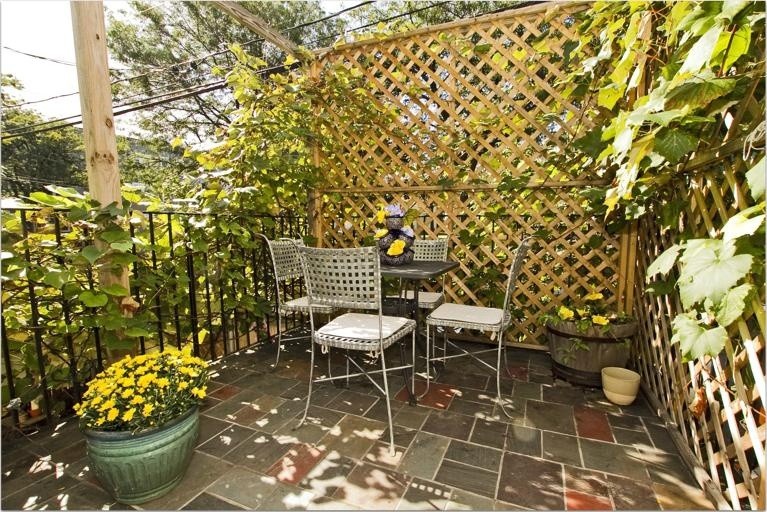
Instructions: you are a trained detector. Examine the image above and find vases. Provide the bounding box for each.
[378,248,414,266]
[379,230,415,247]
[601,366,641,406]
[545,312,638,387]
[83,405,201,503]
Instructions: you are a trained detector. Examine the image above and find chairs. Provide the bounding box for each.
[257,233,341,384]
[384,234,450,365]
[414,236,533,421]
[279,238,416,456]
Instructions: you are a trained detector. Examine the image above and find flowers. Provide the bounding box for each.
[402,225,414,238]
[375,228,388,240]
[535,270,640,367]
[387,239,405,256]
[69,345,217,434]
